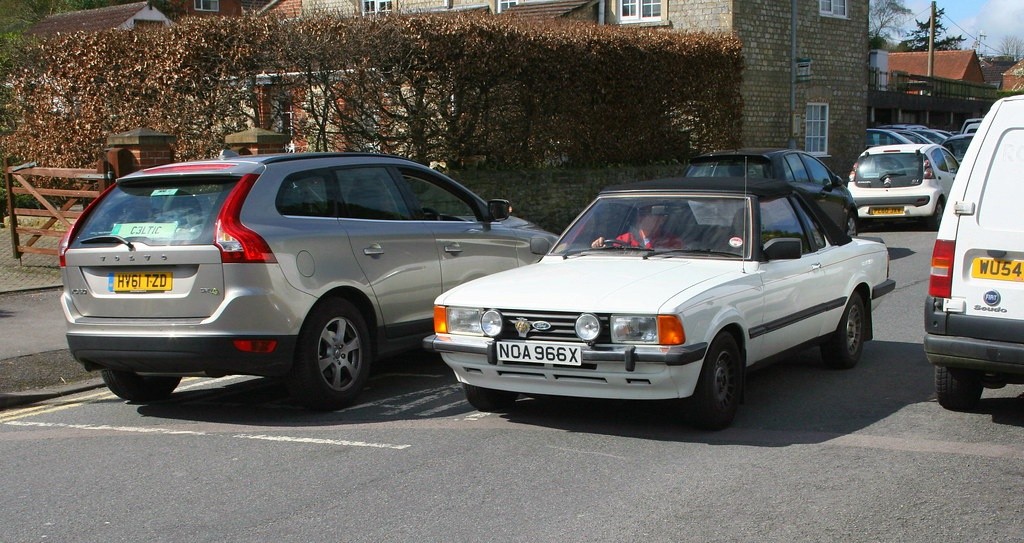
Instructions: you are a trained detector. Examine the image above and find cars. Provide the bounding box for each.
[422,176,896,432]
[681,148,858,237]
[845,124,985,232]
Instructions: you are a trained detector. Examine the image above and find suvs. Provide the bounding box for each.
[56,153,564,412]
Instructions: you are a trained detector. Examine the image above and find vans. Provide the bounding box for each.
[922,95,1024,417]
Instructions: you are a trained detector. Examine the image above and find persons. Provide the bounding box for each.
[591,203,683,250]
[714,206,748,254]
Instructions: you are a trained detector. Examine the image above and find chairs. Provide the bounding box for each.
[713,207,749,257]
[347,173,383,219]
